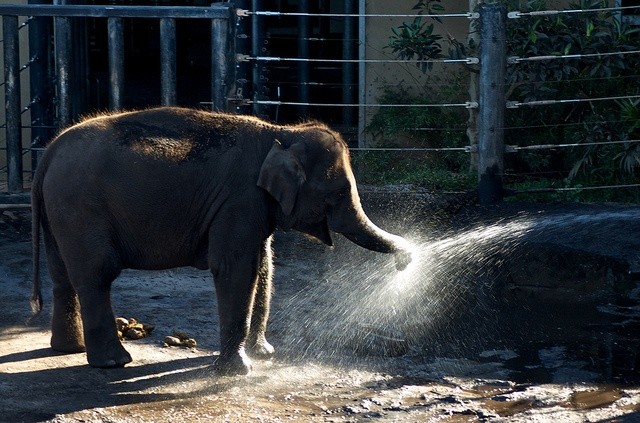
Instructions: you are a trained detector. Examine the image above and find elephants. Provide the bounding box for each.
[29,104,414,376]
